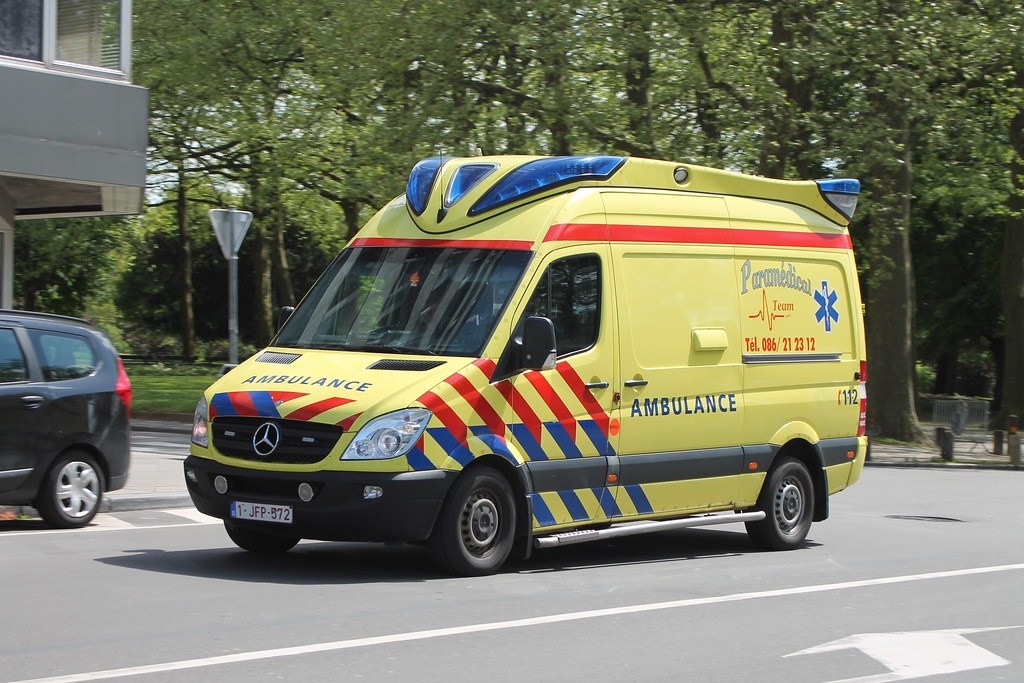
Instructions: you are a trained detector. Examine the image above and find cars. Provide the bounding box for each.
[0,309,133,529]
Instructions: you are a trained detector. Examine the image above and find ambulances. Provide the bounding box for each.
[183,151,869,580]
